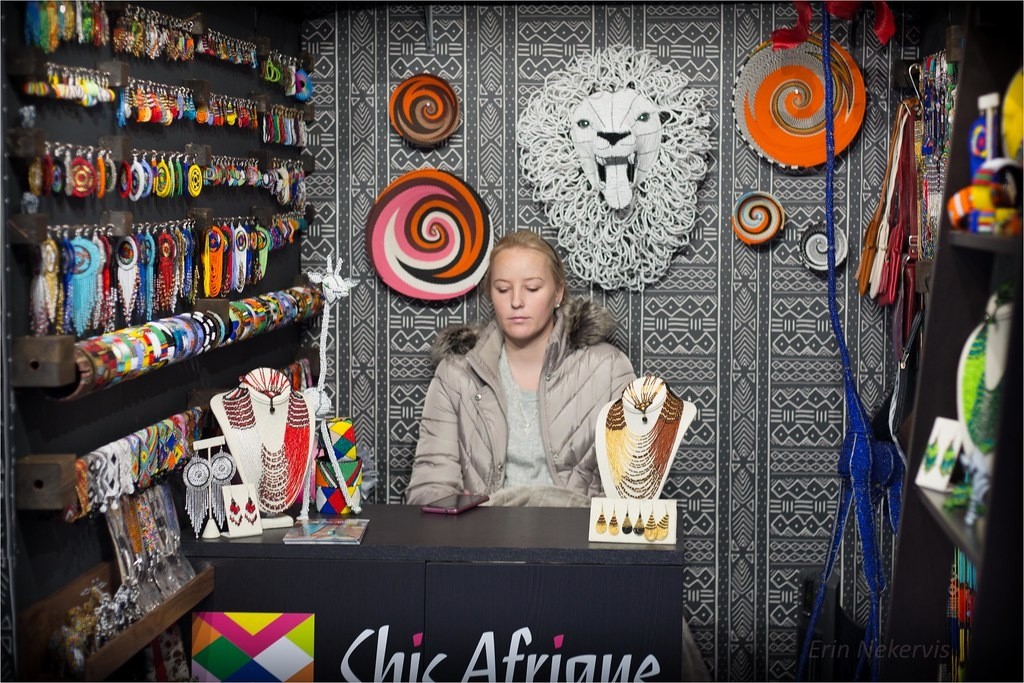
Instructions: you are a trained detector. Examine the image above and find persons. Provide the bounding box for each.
[403,230,637,506]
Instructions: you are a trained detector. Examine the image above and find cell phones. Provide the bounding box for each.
[421,494,489,513]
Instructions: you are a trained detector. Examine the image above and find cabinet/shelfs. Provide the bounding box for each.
[793,0,1023,683]
[0,1,338,674]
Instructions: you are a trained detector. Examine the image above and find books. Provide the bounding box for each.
[282,517,370,545]
